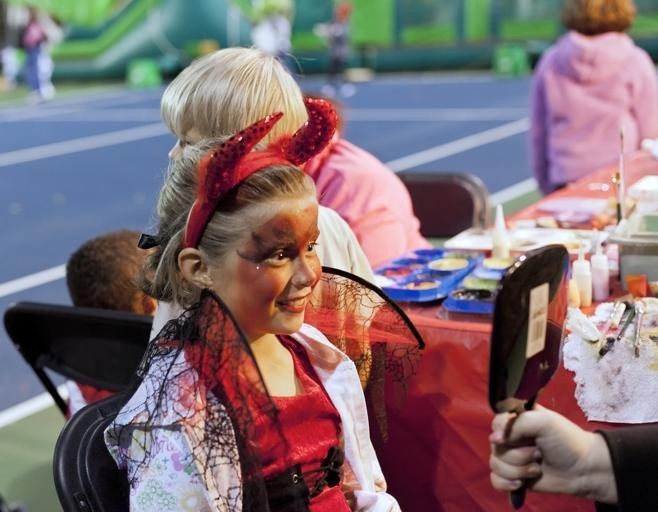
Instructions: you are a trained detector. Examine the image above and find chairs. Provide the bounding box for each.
[50,384,172,511]
[388,167,484,239]
[1,298,155,416]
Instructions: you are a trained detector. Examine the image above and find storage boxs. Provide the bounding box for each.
[608,217,657,293]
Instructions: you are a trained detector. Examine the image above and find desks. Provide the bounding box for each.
[349,149,658,509]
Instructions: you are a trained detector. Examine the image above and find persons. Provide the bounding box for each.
[148,45,385,338]
[487,399,657,511]
[248,1,358,97]
[309,138,434,272]
[101,133,403,511]
[529,0,657,195]
[19,4,64,107]
[65,229,160,422]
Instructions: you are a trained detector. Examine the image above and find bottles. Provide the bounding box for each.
[590,243,610,300]
[573,245,592,307]
[491,203,510,259]
